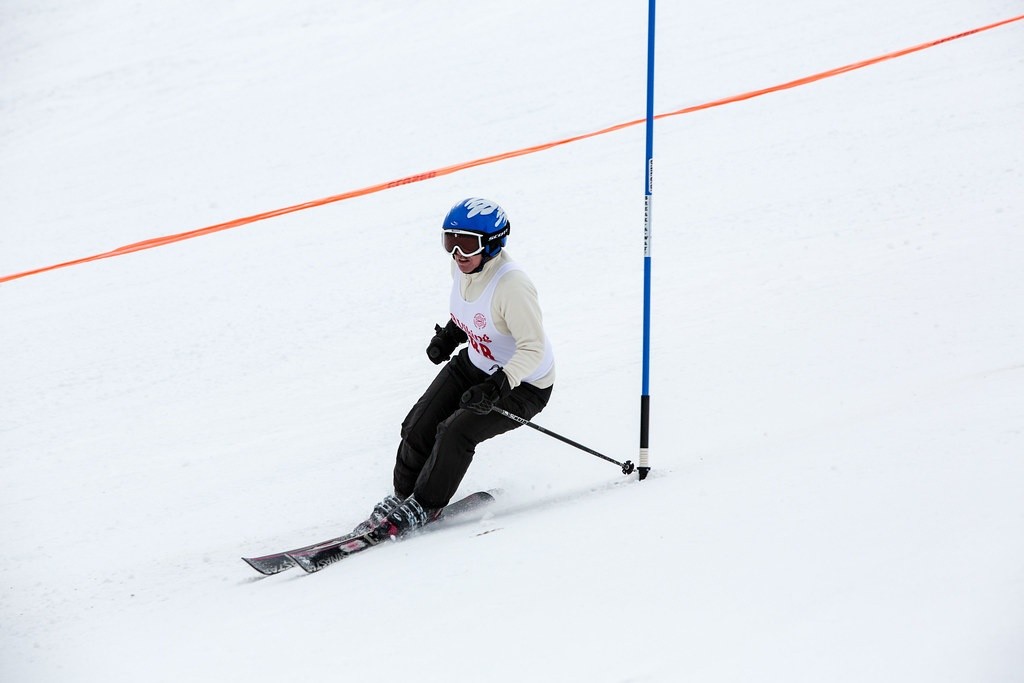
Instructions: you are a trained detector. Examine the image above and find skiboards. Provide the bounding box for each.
[241,491,495,576]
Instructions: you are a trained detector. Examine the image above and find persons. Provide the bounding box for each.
[351,196,556,546]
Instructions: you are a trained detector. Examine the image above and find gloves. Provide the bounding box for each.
[459,366,511,416]
[426,318,468,365]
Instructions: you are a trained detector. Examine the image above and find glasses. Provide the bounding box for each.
[442,220,511,257]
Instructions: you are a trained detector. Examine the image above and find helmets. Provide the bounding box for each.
[443,198,508,274]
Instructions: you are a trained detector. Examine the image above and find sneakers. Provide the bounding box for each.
[369,493,406,528]
[380,492,443,536]
